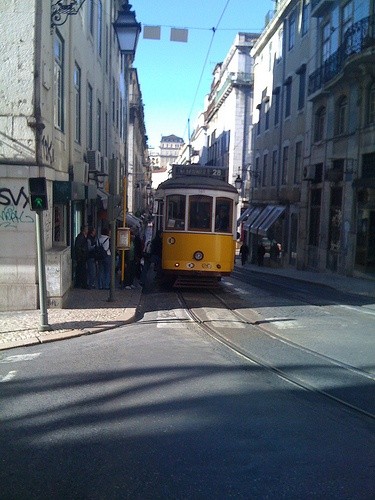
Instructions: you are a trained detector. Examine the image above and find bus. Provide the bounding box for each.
[152,164,240,290]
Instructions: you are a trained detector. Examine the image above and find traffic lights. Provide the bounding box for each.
[28,177,48,212]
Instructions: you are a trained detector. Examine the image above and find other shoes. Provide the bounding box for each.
[125,285,136,289]
[88,287,96,289]
[99,288,110,290]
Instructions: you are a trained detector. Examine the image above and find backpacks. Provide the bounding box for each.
[98,238,108,259]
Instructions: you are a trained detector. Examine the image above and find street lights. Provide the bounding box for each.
[106,1,142,303]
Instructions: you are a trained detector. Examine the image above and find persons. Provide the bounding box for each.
[74,222,150,290]
[267,242,281,261]
[257,243,265,266]
[240,240,248,265]
